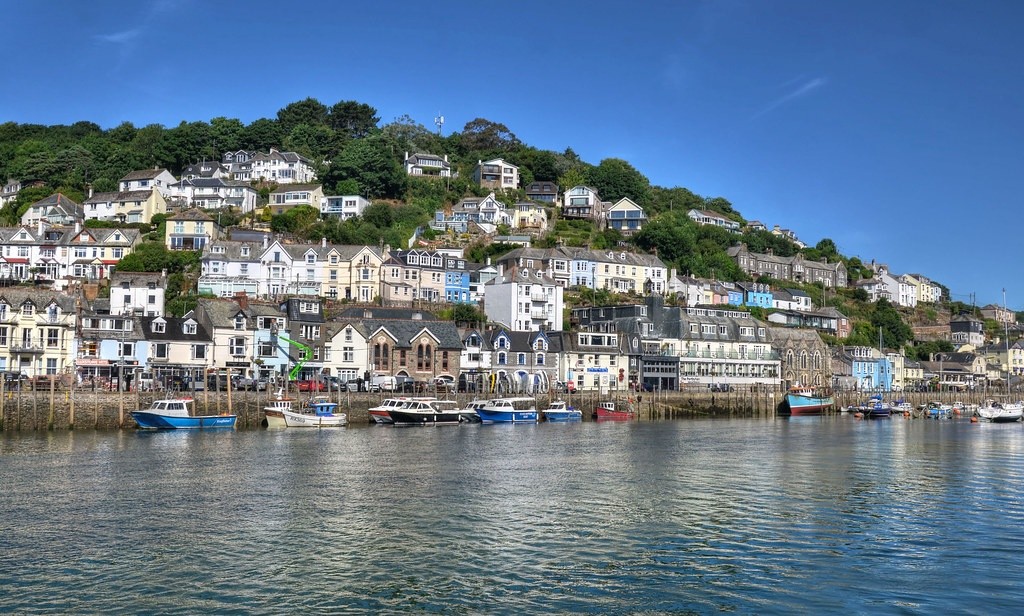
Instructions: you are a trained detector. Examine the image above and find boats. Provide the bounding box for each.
[459,398,582,423]
[594,402,634,421]
[895,402,1024,425]
[387,401,462,427]
[366,396,438,423]
[847,397,894,419]
[783,385,835,416]
[259,389,347,427]
[129,400,238,431]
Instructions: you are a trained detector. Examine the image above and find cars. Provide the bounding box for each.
[550,380,563,392]
[397,380,428,394]
[711,382,734,392]
[751,382,767,393]
[425,377,454,392]
[158,374,188,391]
[889,381,931,394]
[185,372,380,395]
[563,382,577,394]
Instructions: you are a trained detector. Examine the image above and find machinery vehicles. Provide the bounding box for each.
[273,333,314,382]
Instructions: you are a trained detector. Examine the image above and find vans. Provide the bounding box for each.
[371,374,397,392]
